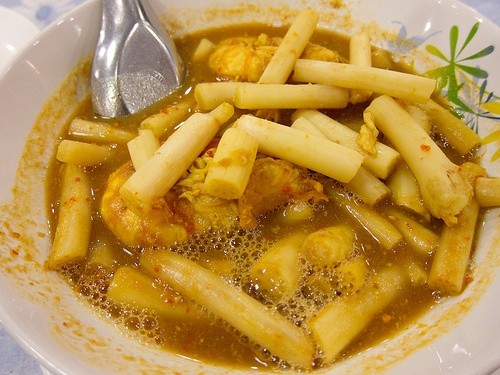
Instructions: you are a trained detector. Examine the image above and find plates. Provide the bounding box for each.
[1,1,499,373]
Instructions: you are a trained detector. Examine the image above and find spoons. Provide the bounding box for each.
[91,1,187,117]
